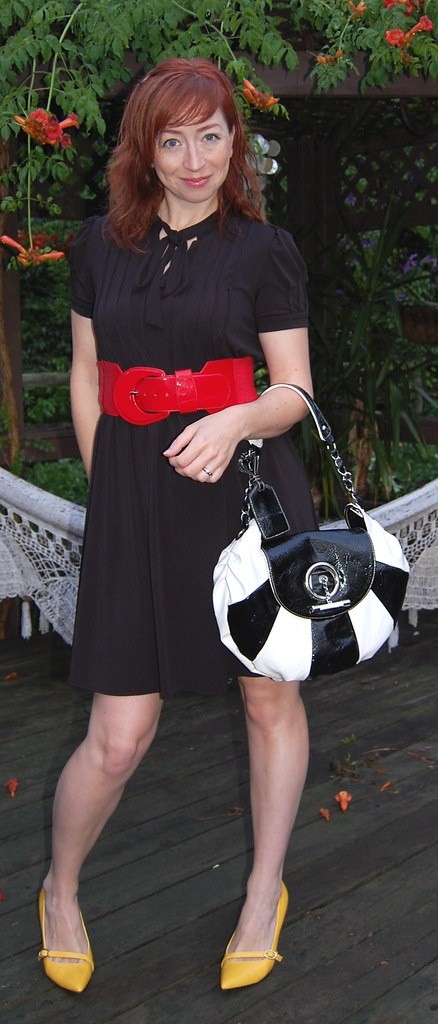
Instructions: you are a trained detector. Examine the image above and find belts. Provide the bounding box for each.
[96,353,259,427]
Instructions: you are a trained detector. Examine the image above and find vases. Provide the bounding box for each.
[0,463,437,673]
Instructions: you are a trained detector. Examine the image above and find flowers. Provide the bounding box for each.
[6,187,438,531]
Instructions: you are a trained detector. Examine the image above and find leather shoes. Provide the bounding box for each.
[39,886,94,993]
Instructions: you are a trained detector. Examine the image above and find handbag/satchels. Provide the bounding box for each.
[213,386,410,683]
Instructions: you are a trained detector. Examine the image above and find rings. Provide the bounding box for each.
[202,467,213,476]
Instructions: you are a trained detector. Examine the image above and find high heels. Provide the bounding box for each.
[220,879,289,990]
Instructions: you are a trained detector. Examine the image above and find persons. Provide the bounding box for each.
[38,56,315,993]
